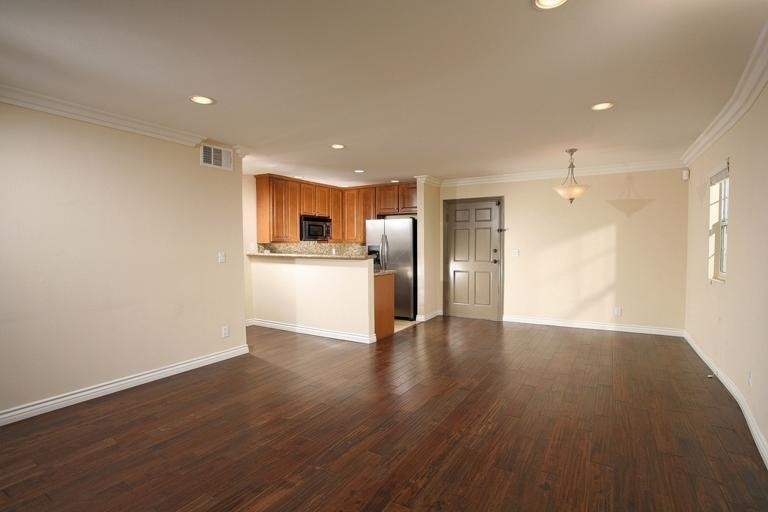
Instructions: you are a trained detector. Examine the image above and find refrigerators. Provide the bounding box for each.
[365,218,417,320]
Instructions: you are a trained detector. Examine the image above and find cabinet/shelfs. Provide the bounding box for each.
[254,173,301,244]
[343,186,376,244]
[300,180,330,217]
[375,183,417,215]
[330,185,343,243]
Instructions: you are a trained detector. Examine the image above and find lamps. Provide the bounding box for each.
[552,149,590,206]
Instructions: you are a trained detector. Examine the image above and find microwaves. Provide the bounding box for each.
[300,221,332,240]
